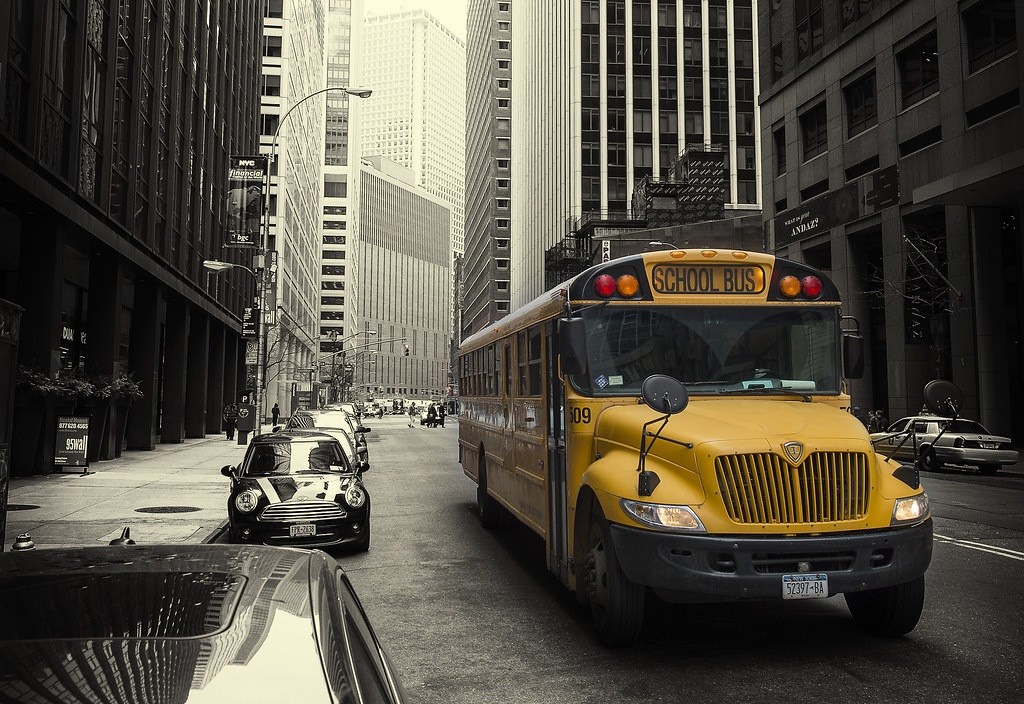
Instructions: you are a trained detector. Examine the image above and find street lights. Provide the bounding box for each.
[649,242,679,250]
[442,369,456,415]
[341,350,376,402]
[203,87,372,437]
[330,330,376,404]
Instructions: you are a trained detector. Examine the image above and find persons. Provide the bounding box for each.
[853,407,866,428]
[272,403,280,426]
[223,400,238,440]
[876,409,888,432]
[868,409,879,434]
[365,399,446,428]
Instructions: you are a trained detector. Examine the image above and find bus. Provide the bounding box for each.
[457,249,934,649]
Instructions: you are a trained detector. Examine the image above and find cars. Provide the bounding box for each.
[421,405,441,425]
[0,526,409,703]
[321,402,371,469]
[869,416,1019,474]
[221,428,370,552]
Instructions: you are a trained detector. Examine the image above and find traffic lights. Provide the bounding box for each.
[401,343,410,358]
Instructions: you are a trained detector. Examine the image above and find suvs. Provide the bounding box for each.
[272,407,366,453]
